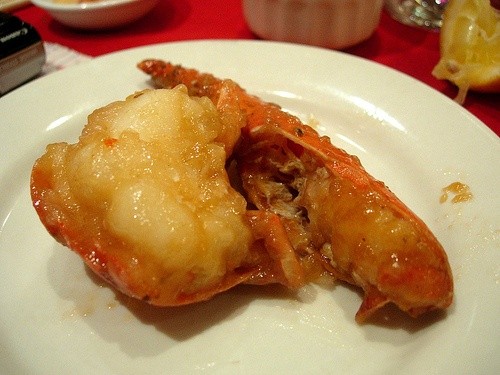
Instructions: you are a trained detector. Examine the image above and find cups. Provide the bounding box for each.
[385,0,445,30]
[242,0,384,50]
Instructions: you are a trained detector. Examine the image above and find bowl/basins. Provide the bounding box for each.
[30,0,159,30]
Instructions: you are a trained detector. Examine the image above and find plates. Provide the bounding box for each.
[1,39,499,373]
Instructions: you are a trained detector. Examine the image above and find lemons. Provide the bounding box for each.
[431,0,500,103]
